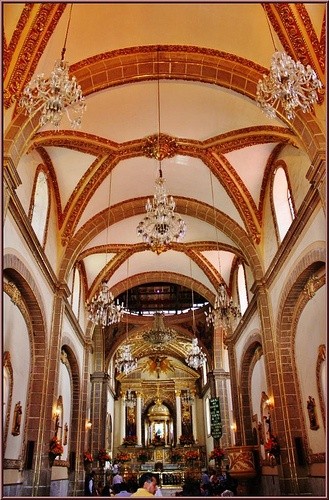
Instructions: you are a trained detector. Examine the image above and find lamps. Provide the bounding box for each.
[16,4,89,135]
[83,172,127,327]
[141,311,176,344]
[136,49,187,252]
[253,4,321,118]
[183,335,207,369]
[113,343,136,374]
[206,168,246,332]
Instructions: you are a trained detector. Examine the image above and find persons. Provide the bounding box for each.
[99,468,138,497]
[84,471,97,497]
[130,472,158,497]
[151,475,162,497]
[200,470,236,496]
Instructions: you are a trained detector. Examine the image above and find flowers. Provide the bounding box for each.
[184,451,198,461]
[84,451,98,468]
[47,436,63,467]
[100,448,112,467]
[208,447,225,462]
[264,439,280,457]
[117,453,130,461]
